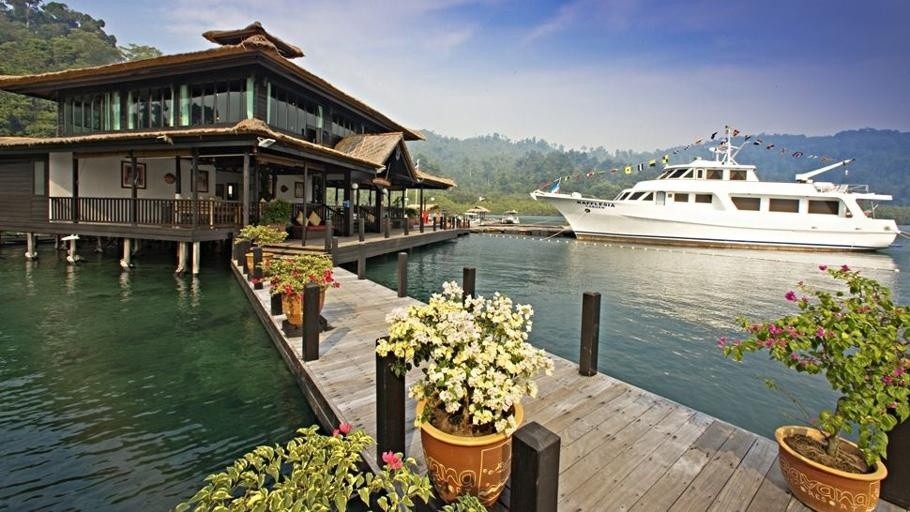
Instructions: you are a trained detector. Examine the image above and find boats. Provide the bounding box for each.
[529,125,902,252]
[502,207,521,224]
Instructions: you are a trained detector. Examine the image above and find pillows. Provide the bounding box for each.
[296,211,322,227]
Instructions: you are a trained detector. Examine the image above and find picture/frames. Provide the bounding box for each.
[190,168,209,193]
[294,181,305,198]
[120,160,148,189]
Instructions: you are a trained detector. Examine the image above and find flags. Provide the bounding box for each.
[732,129,762,146]
[542,174,575,192]
[767,144,803,159]
[674,131,717,154]
[638,154,669,171]
[577,165,631,179]
[807,155,830,162]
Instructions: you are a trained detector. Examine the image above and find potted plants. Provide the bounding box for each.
[233,199,293,276]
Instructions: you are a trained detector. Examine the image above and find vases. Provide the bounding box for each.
[281,286,329,328]
[414,398,524,506]
[774,424,887,512]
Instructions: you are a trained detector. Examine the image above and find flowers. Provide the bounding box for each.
[717,265,910,469]
[255,253,340,297]
[374,280,556,439]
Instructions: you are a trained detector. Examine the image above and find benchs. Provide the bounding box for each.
[294,225,336,239]
